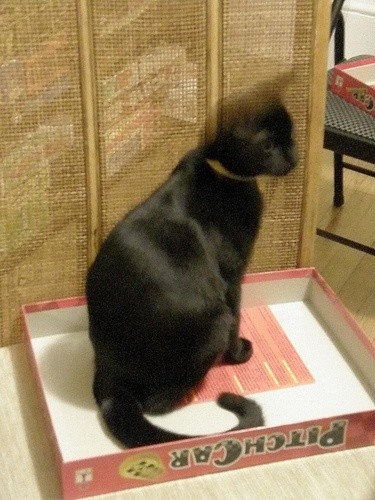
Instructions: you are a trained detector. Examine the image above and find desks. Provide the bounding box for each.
[0,340,375,500]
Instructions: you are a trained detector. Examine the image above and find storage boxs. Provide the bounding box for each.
[330,56,375,115]
[20,267,375,500]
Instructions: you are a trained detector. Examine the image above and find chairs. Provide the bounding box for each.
[323,0,375,209]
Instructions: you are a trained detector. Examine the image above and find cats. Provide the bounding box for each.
[85,69,297,452]
